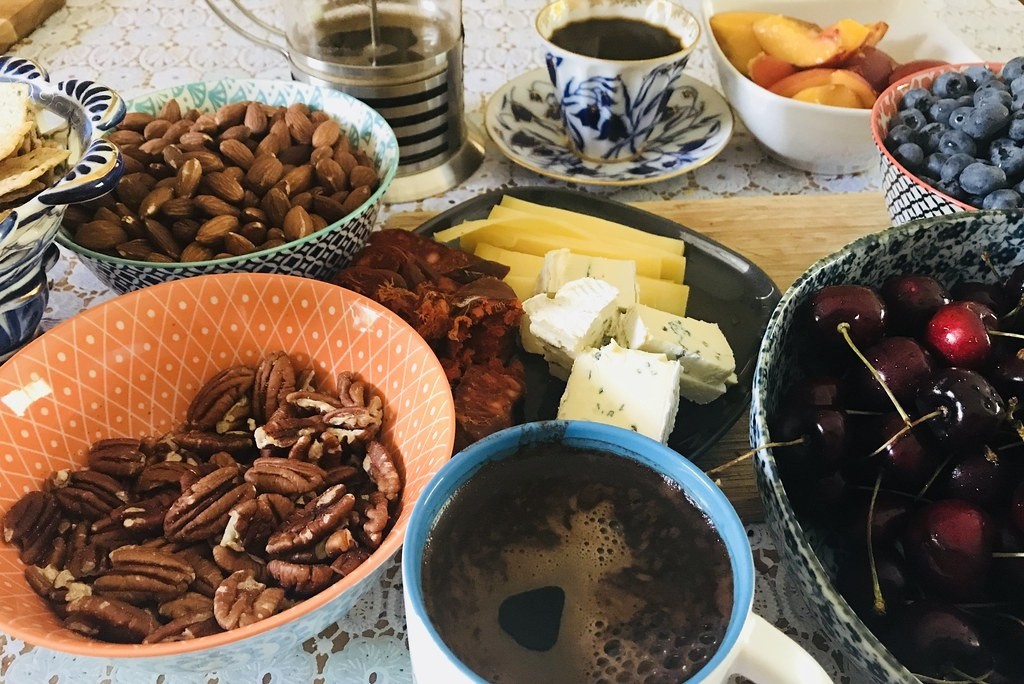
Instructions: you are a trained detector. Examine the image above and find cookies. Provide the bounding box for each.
[0,80,73,212]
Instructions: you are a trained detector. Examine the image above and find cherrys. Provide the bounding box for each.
[700,250,1024,684]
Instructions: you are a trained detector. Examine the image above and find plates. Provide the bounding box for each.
[484,65,736,187]
[410,186,784,461]
[719,612,835,684]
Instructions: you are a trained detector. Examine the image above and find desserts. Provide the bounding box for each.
[516,248,737,448]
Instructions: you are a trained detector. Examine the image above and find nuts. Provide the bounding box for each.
[59,97,377,262]
[2,348,400,644]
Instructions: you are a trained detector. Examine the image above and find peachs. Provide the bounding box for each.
[708,11,952,109]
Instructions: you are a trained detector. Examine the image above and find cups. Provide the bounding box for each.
[400,420,834,684]
[536,0,701,164]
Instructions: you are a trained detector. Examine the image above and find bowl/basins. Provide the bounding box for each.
[57,78,400,297]
[869,61,1008,227]
[701,0,983,175]
[747,208,1024,684]
[1,273,456,673]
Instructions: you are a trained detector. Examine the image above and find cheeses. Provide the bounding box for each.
[435,193,689,318]
